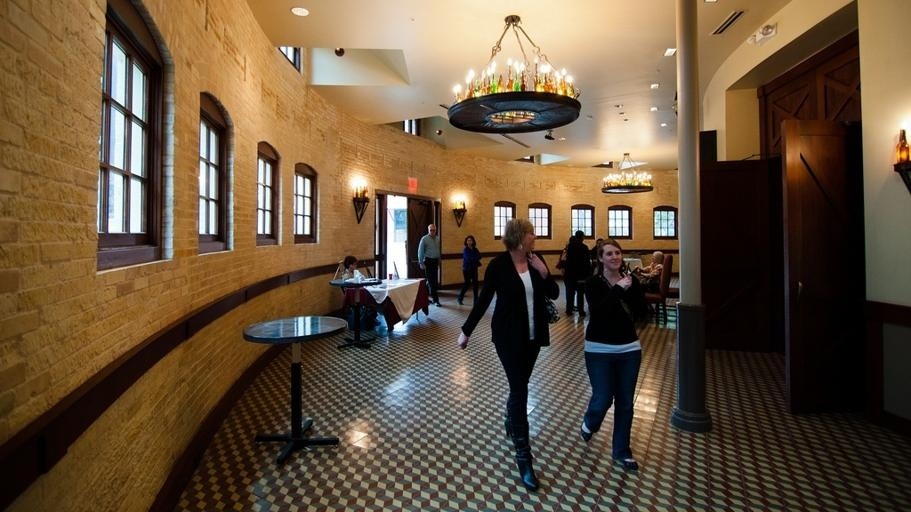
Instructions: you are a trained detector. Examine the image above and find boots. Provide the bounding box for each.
[505,392,515,442]
[513,433,540,492]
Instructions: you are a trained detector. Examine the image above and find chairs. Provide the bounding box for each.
[647,255,675,324]
[340,287,368,328]
[655,289,680,315]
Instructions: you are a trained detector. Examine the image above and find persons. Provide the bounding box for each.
[563,230,591,317]
[456,235,483,305]
[555,236,578,311]
[590,238,603,266]
[456,218,560,492]
[634,250,664,294]
[579,239,647,471]
[341,256,381,325]
[418,224,442,307]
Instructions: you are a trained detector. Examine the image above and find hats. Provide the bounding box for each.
[575,231,587,237]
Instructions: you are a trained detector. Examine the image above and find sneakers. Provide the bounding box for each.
[456,296,464,305]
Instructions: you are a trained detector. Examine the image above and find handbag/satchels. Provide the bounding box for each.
[478,260,482,267]
[556,258,566,270]
[545,297,562,324]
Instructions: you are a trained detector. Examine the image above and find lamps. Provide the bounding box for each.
[448,14,582,134]
[894,127,911,193]
[452,193,467,227]
[350,175,370,224]
[599,151,653,194]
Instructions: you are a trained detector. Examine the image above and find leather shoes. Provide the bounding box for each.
[580,421,593,441]
[433,299,443,307]
[614,459,640,471]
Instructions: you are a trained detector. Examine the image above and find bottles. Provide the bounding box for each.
[896,129,911,163]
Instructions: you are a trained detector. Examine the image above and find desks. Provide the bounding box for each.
[344,279,429,333]
[332,280,383,349]
[241,316,349,466]
